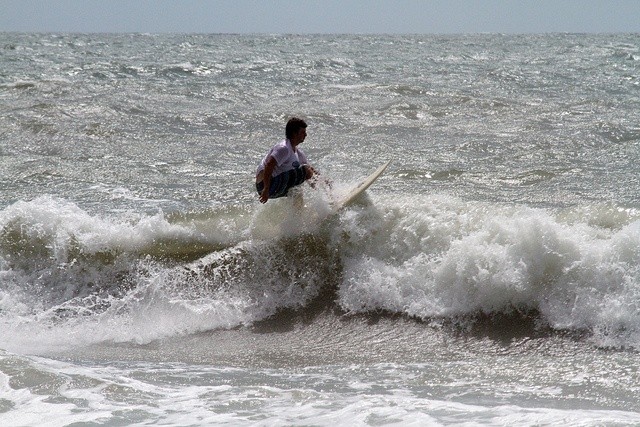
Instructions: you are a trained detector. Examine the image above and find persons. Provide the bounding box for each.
[256,117,332,205]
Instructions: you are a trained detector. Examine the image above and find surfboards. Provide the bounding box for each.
[337,158,392,210]
